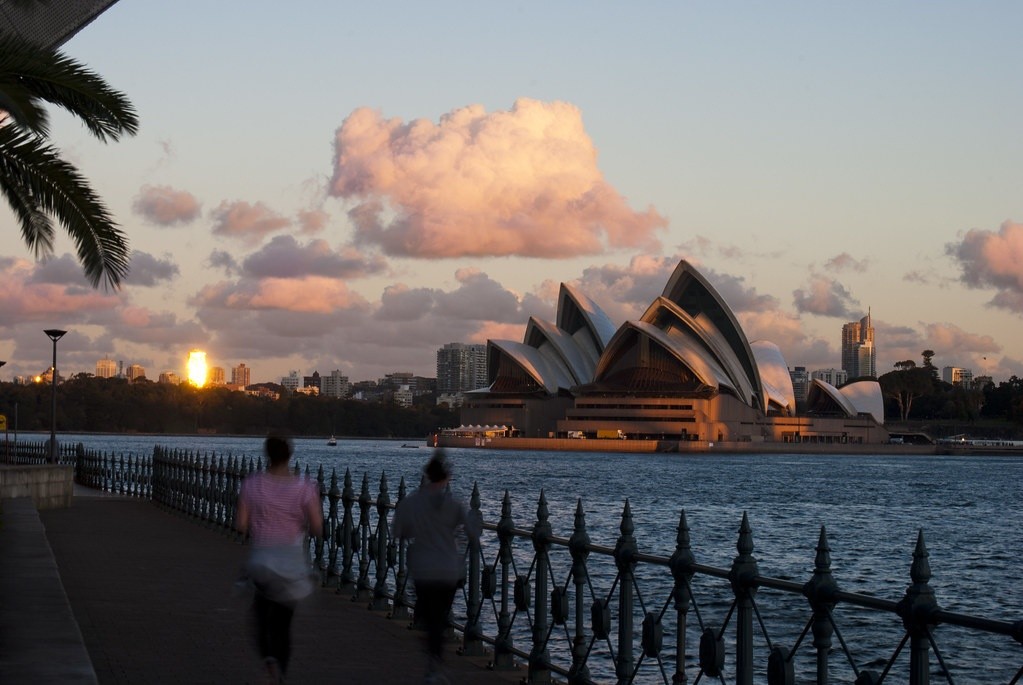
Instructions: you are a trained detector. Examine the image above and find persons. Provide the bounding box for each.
[390,448,471,685]
[233,429,324,685]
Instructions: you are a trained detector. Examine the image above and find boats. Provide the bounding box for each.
[328,435,338,447]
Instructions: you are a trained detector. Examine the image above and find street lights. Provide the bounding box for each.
[43,328,68,466]
